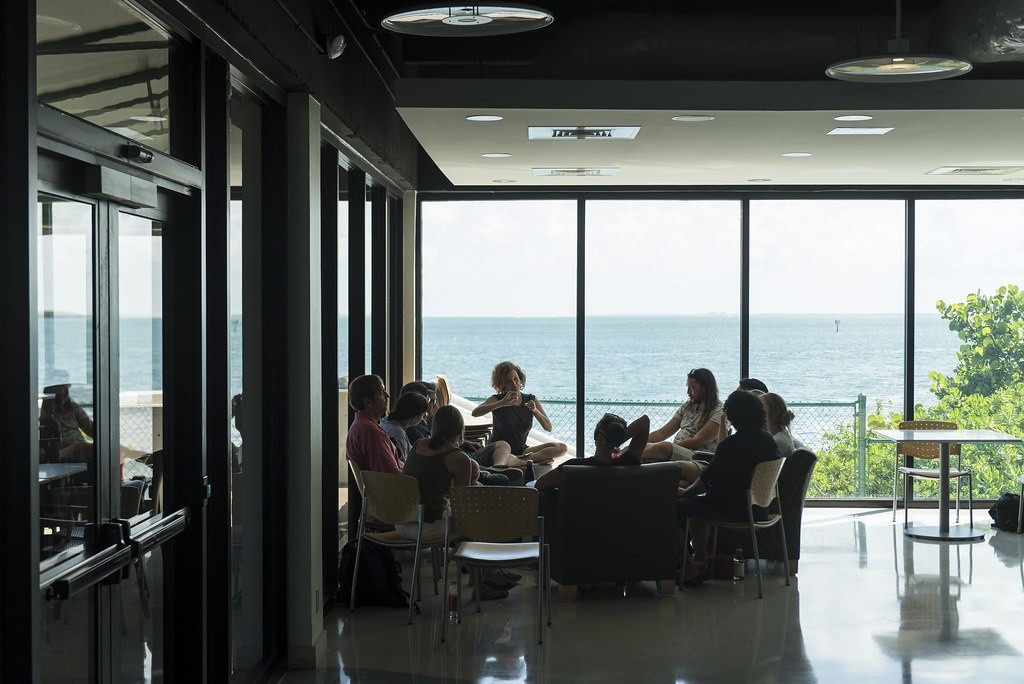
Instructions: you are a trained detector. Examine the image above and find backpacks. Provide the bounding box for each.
[323,538,421,616]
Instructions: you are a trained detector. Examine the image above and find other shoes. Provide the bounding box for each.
[485,570,515,590]
[503,571,522,582]
[472,581,509,600]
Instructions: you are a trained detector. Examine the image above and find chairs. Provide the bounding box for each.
[679,455,791,601]
[894,422,975,530]
[536,459,683,602]
[434,375,494,448]
[348,469,472,625]
[37,475,153,619]
[707,447,818,578]
[345,458,439,615]
[439,485,552,643]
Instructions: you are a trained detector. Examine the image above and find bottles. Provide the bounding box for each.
[448,582,458,621]
[733,548,744,581]
[523,460,535,484]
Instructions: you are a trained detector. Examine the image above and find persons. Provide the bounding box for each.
[471,361,563,466]
[350,374,522,601]
[39,385,160,520]
[674,377,795,585]
[621,368,733,461]
[535,412,650,589]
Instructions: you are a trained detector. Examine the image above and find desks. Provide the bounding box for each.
[871,428,1023,545]
[38,463,88,491]
[869,535,1024,663]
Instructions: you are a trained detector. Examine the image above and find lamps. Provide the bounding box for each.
[375,0,555,40]
[123,144,154,163]
[825,0,973,85]
[326,32,348,61]
